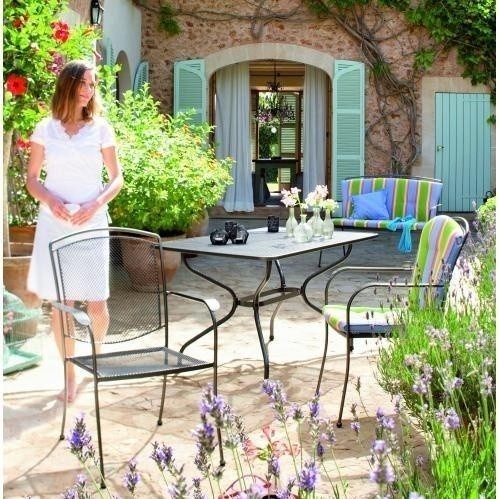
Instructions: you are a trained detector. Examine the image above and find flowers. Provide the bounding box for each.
[279,184,339,240]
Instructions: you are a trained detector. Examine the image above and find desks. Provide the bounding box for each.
[151,226,380,389]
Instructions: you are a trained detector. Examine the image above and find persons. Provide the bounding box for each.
[24,58,127,405]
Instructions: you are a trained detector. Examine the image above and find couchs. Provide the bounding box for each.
[317,174,444,268]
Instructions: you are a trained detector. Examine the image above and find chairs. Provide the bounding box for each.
[48,227,226,486]
[314,213,471,429]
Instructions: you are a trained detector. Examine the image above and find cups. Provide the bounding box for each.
[267,214,280,232]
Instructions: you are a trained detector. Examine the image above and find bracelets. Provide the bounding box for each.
[95,196,104,205]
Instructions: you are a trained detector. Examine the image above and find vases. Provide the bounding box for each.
[293,214,313,242]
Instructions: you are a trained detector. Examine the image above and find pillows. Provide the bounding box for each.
[348,190,390,219]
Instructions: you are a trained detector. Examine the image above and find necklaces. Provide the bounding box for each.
[63,121,85,136]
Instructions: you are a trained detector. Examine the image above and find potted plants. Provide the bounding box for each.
[93,63,238,294]
